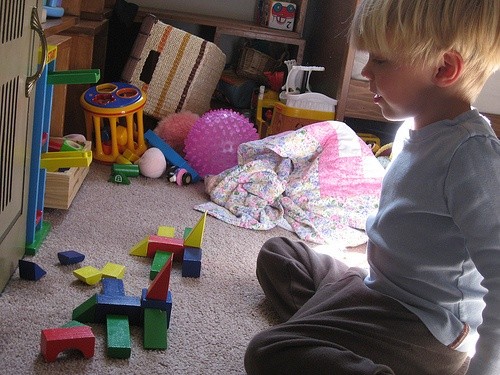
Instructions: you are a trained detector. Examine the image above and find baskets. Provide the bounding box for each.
[119,13,227,123]
[237,41,288,89]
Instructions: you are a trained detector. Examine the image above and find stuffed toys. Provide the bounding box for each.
[136,111,203,179]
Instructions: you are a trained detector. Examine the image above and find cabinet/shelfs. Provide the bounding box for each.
[1,1,49,296]
[304,0,499,141]
[110,0,308,81]
[41,1,116,209]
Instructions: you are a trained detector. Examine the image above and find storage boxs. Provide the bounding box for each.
[261,0,296,32]
[121,14,227,120]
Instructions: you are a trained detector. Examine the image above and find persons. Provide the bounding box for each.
[244,0,500,375]
